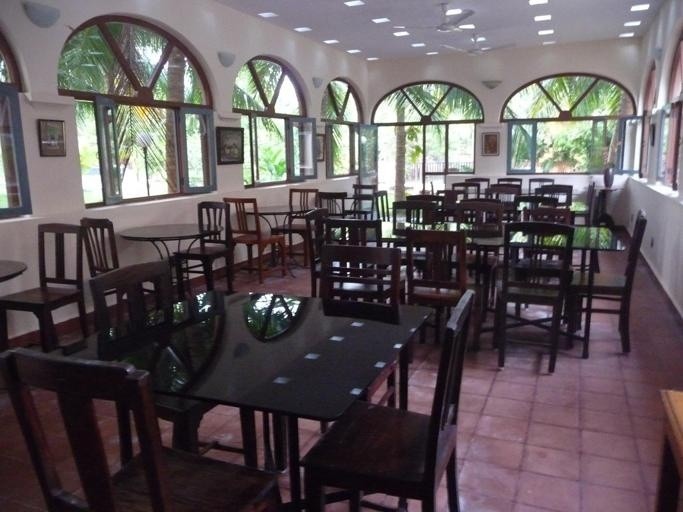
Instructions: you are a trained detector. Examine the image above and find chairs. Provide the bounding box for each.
[90,259,254,469]
[3,348,284,511]
[3,177,648,430]
[302,289,475,511]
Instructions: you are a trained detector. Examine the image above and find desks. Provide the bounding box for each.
[46,290,436,512]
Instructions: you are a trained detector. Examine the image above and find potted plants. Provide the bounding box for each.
[604,163,616,188]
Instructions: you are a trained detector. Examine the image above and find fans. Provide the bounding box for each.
[439,33,517,57]
[405,2,476,33]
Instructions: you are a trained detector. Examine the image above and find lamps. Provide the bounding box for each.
[481,80,502,89]
[311,77,324,88]
[218,52,235,68]
[24,2,60,28]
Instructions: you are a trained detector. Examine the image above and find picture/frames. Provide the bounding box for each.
[38,119,66,157]
[315,134,326,162]
[216,127,244,165]
[481,131,500,156]
